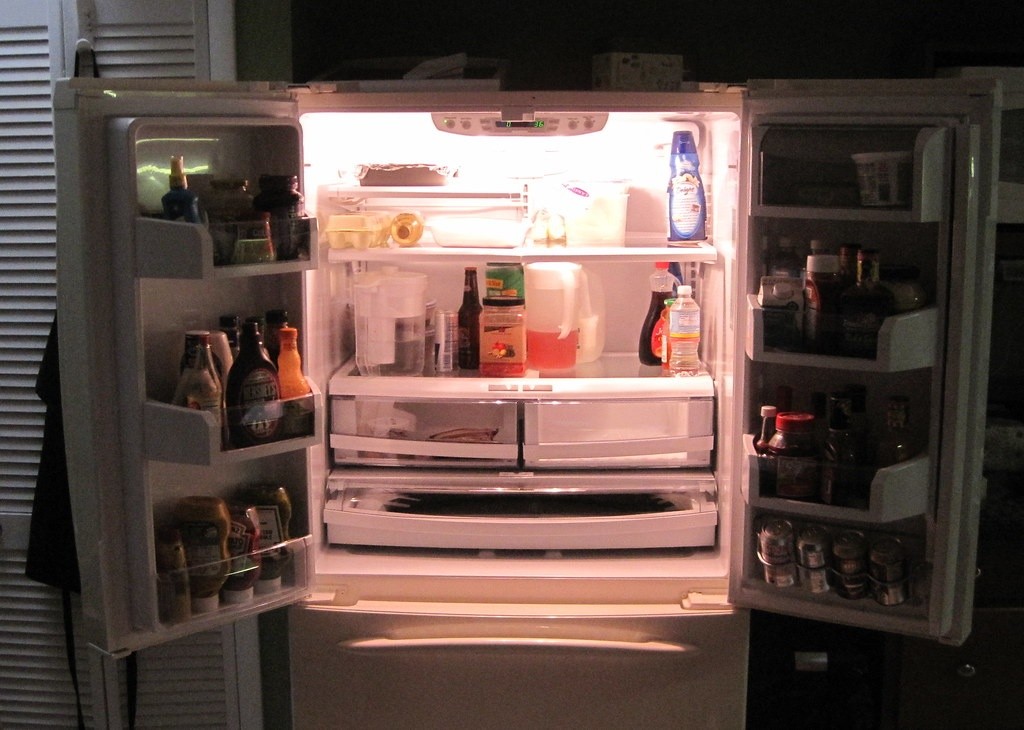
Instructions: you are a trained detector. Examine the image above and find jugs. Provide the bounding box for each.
[523,261,581,372]
[352,272,428,377]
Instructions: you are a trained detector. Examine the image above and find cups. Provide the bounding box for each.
[851,151,900,208]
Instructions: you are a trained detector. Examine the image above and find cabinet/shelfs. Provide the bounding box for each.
[328,248,717,401]
[139,211,326,462]
[742,121,954,521]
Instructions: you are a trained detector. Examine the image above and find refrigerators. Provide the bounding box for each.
[54,65,1004,728]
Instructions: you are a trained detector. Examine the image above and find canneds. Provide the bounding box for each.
[661,298,678,376]
[767,411,819,499]
[432,309,458,373]
[760,518,908,605]
[206,174,305,264]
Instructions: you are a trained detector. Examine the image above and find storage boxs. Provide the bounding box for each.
[593,50,683,90]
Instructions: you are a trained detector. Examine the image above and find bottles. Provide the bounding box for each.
[157,481,292,623]
[662,298,677,372]
[771,235,927,360]
[161,153,306,265]
[664,129,707,247]
[390,211,423,247]
[670,286,700,377]
[637,262,681,366]
[756,384,916,510]
[458,267,484,370]
[173,313,316,445]
